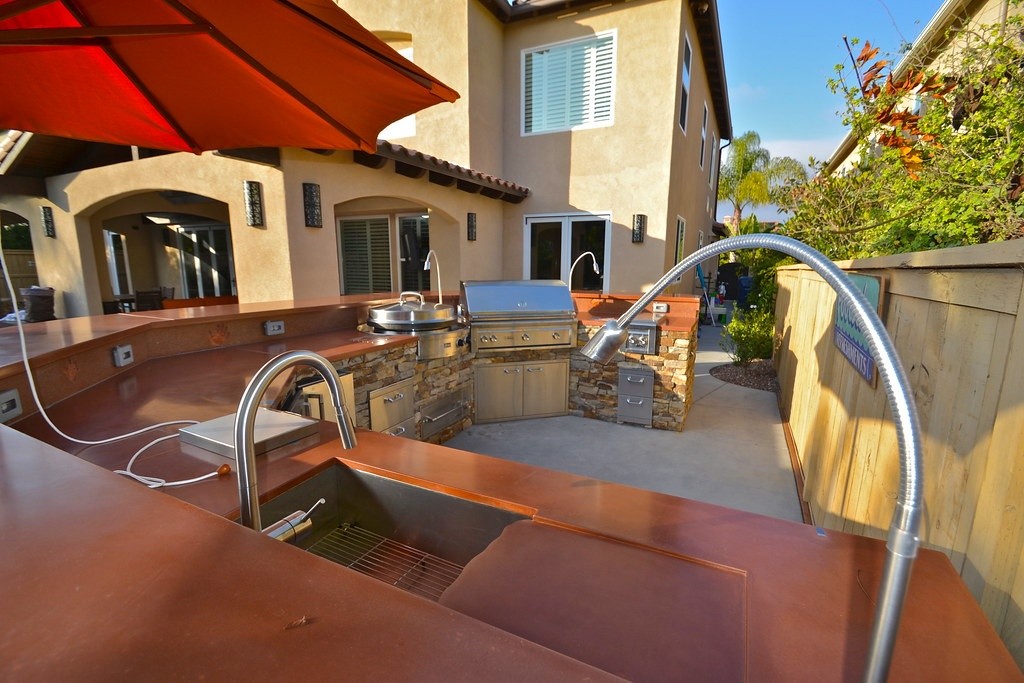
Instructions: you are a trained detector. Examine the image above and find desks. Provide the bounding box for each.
[114,294,135,313]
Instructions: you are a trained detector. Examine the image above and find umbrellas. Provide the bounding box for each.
[0,0,461,156]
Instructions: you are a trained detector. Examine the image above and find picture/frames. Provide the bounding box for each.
[835,271,884,388]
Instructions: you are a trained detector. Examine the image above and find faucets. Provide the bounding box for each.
[567,250,600,293]
[424,250,444,307]
[233,350,359,543]
[579,234,925,683]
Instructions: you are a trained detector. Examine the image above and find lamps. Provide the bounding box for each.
[303,183,323,228]
[632,214,645,244]
[243,180,264,227]
[39,206,54,237]
[424,250,443,305]
[568,251,599,292]
[467,213,476,241]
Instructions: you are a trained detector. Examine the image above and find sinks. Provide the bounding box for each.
[220,458,528,603]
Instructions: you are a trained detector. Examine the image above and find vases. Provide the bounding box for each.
[19,288,55,322]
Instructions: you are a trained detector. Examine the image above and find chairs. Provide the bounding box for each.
[135,286,174,313]
